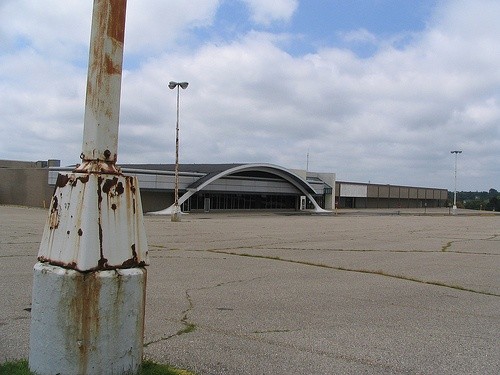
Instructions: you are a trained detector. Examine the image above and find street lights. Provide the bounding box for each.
[167,81,190,222]
[450,150,464,210]
